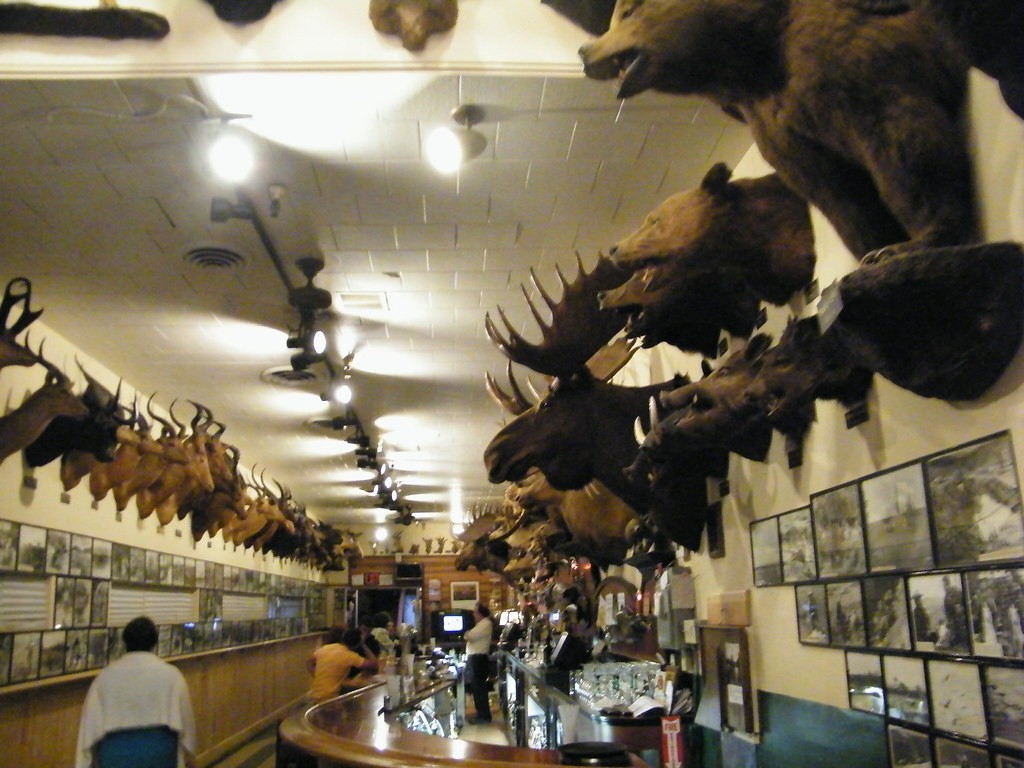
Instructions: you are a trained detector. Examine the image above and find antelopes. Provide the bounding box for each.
[155,398,255,541]
[223,461,299,555]
[0,330,188,512]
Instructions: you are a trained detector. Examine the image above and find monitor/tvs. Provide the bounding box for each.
[394,561,422,580]
[439,613,466,635]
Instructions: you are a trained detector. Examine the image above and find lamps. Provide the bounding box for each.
[199,121,261,179]
[288,261,414,525]
[211,197,253,222]
[430,104,487,171]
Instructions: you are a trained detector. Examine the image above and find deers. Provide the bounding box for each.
[484,251,712,552]
[455,500,527,586]
[0,275,44,374]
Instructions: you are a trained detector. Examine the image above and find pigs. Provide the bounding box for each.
[624,241,1024,491]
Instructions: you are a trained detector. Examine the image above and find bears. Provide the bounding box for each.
[597,162,822,358]
[577,0,984,263]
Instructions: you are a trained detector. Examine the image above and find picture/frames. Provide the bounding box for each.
[0,518,326,687]
[749,427,1024,768]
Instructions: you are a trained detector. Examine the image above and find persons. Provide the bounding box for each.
[307,612,421,701]
[75,615,198,768]
[807,473,1024,660]
[463,603,492,726]
